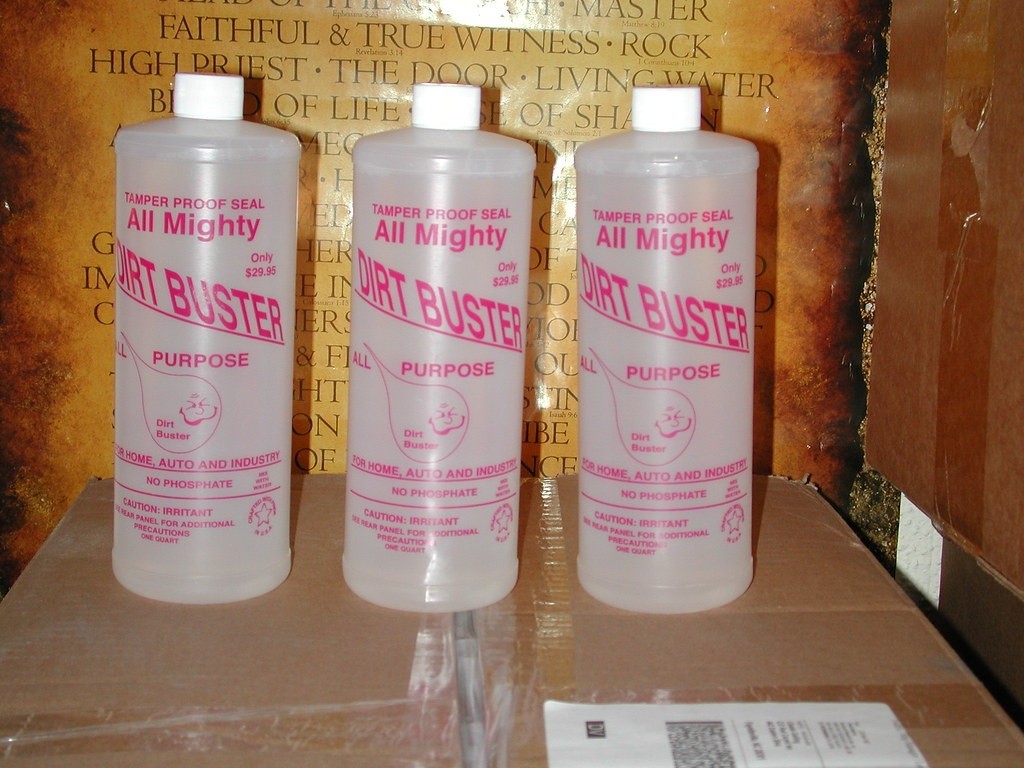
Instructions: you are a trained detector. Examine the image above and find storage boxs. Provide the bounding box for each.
[0,473,1024,768]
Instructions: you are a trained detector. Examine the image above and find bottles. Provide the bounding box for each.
[574,87,753,614]
[341,83,536,613]
[112,74,300,605]
